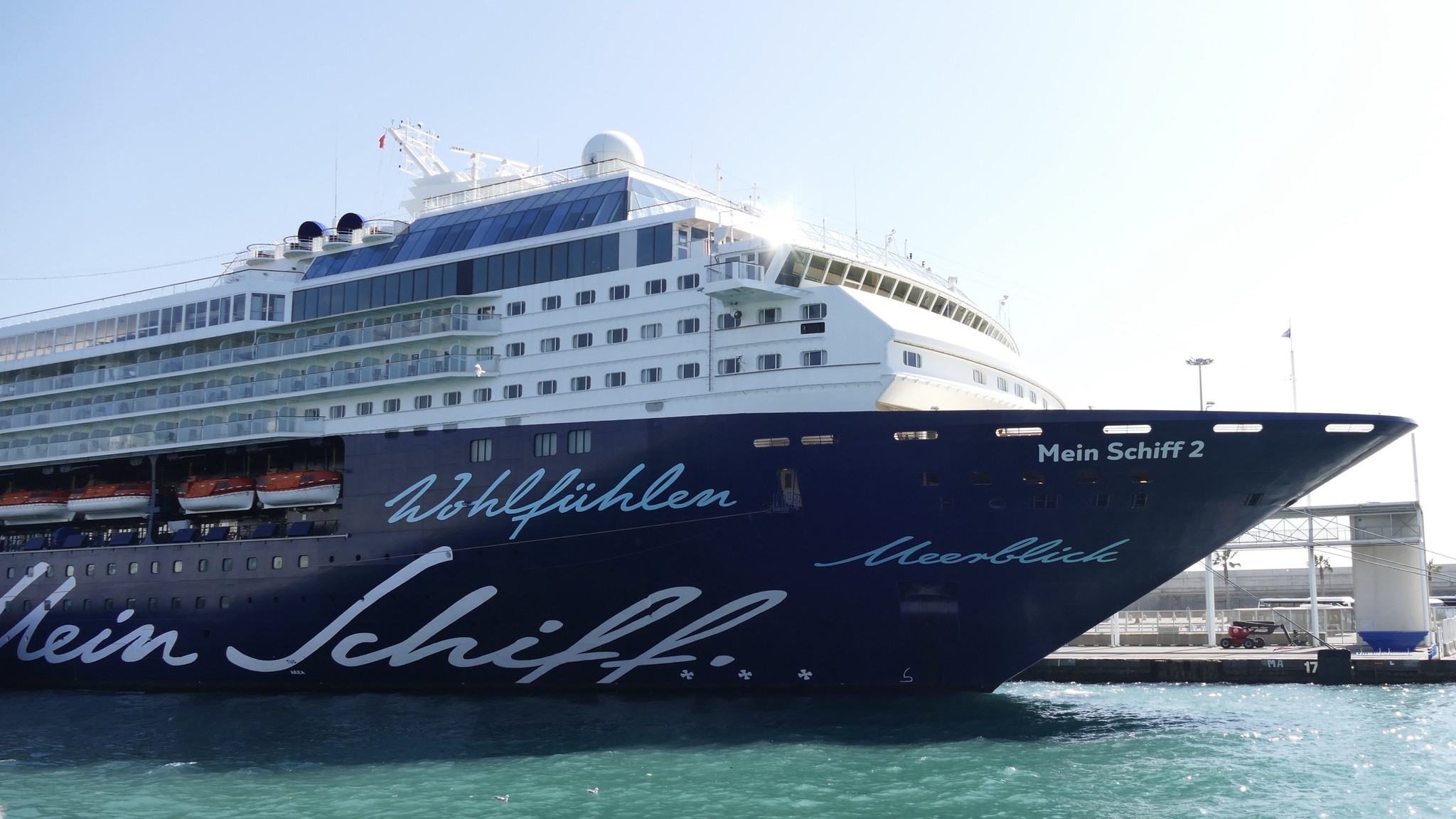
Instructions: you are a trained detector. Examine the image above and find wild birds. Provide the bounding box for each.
[473,363,485,378]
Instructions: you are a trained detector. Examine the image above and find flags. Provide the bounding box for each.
[1282,328,1290,337]
[379,135,385,148]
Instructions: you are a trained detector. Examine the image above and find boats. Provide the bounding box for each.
[168,471,256,514]
[257,470,344,511]
[67,477,162,521]
[0,118,1424,699]
[0,489,78,529]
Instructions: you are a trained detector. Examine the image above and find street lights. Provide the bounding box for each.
[1183,359,1220,651]
